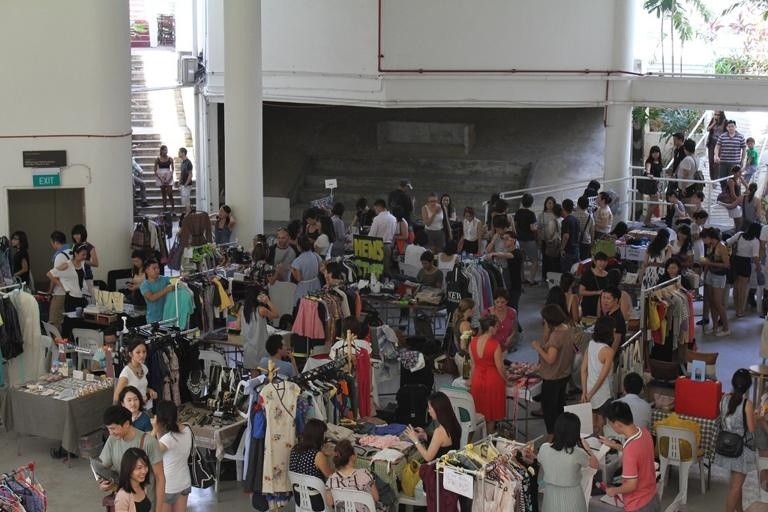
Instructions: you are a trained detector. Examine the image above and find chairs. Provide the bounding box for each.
[10,212,766,511]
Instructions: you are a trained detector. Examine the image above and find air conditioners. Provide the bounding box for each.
[173,47,193,84]
[180,55,201,87]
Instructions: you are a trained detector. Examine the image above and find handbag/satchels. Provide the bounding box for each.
[186,446,216,488]
[709,237,731,275]
[731,233,745,264]
[654,206,660,218]
[103,489,116,511]
[715,431,743,458]
[694,170,705,192]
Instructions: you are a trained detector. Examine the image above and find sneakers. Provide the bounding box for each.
[696,319,731,337]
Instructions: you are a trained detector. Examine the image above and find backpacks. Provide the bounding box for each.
[395,383,432,431]
